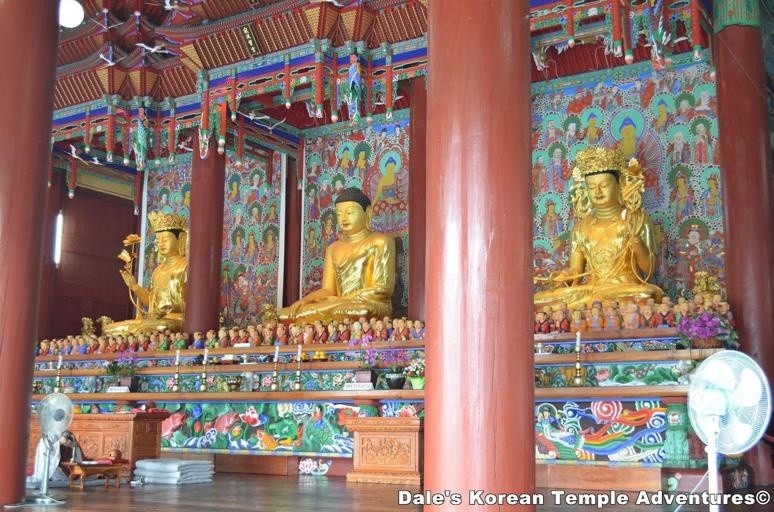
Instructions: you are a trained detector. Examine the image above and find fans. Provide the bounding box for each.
[35,392,75,506]
[687,348,773,511]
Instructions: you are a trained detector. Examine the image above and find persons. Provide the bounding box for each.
[34,53,735,356]
[35,430,88,482]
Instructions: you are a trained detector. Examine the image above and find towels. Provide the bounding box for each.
[133,457,214,486]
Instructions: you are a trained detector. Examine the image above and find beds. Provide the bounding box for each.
[61,460,130,491]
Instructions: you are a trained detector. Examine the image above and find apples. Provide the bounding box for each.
[314,351,327,359]
